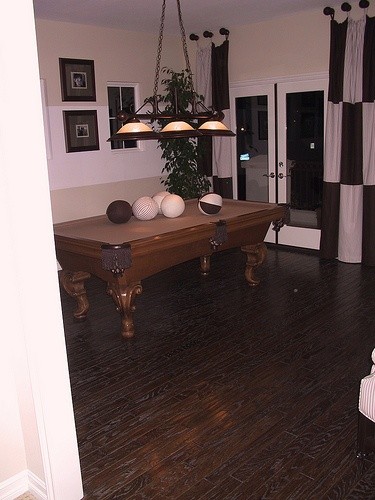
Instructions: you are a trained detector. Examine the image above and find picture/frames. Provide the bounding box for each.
[258,110,267,140]
[257,95,268,106]
[62,110,100,153]
[59,58,97,102]
[300,111,317,137]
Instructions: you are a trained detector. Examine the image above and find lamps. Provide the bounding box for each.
[105,0,237,141]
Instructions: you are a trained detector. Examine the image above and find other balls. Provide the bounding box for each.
[197,192,223,215]
[152,192,170,215]
[132,196,158,220]
[161,195,185,218]
[106,199,131,224]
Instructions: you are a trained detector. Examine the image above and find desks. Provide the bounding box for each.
[53,197,286,339]
